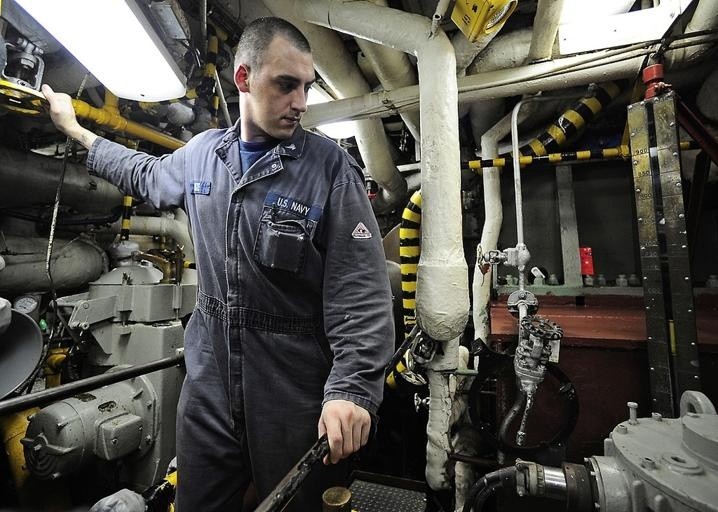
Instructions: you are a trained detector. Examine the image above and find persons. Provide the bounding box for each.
[38,14,398,511]
